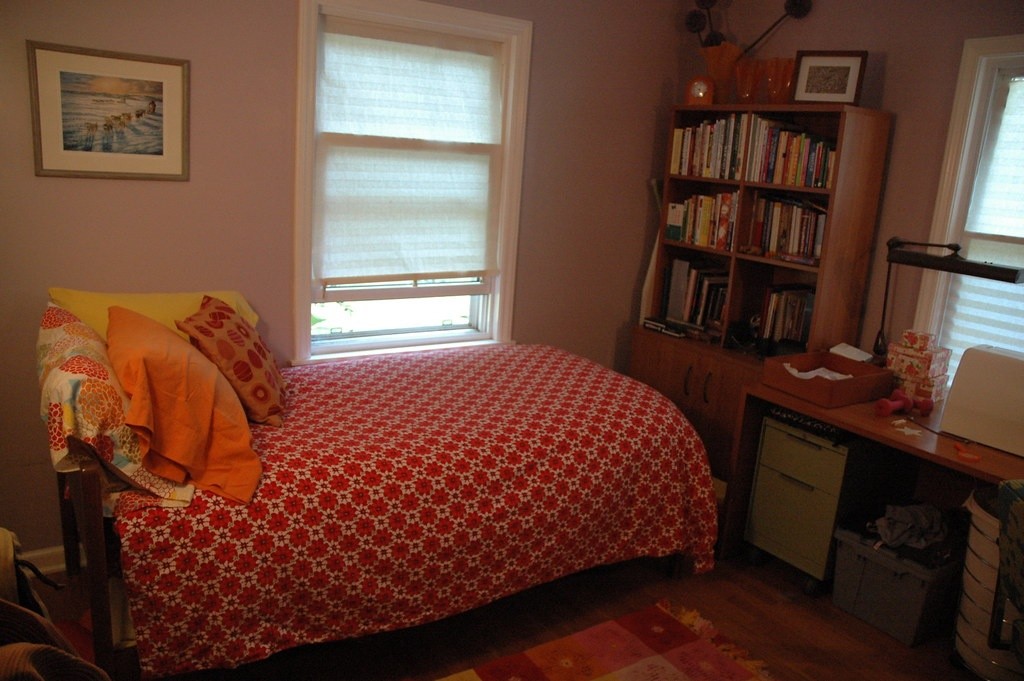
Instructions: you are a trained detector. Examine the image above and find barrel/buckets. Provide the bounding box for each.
[953,488,1024,681]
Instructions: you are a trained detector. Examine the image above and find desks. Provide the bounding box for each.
[714,379,1024,563]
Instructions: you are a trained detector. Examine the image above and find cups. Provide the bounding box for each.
[736,62,753,104]
[766,57,793,105]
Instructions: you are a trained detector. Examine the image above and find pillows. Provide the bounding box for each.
[175,295,287,428]
[107,305,263,505]
[49,286,260,344]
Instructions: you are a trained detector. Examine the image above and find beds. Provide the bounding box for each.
[55,343,721,681]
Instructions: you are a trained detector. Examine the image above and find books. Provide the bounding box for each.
[661,255,813,352]
[666,112,836,267]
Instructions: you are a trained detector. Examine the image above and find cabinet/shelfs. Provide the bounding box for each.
[740,413,922,596]
[628,104,895,483]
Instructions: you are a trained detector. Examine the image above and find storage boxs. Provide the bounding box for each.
[885,343,954,378]
[898,375,951,404]
[899,328,940,351]
[830,525,966,647]
[762,352,897,408]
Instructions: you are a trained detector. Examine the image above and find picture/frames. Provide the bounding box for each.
[786,49,868,108]
[25,39,191,183]
